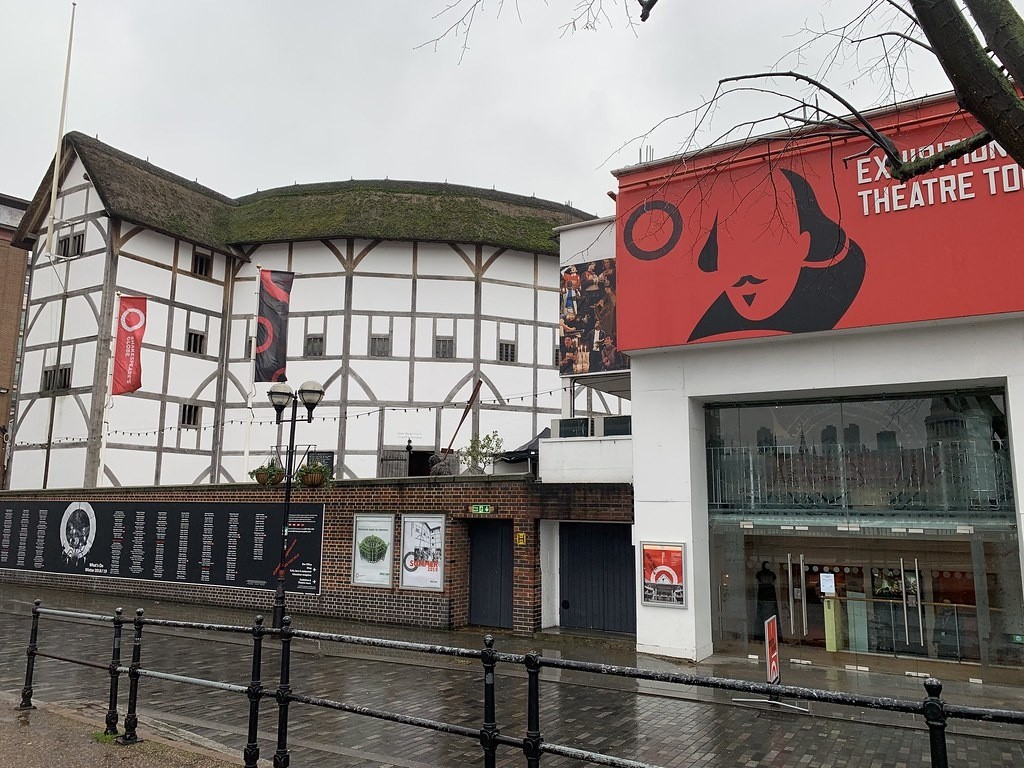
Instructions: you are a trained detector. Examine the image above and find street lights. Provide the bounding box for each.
[266,381,327,644]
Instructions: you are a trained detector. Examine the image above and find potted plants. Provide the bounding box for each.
[294,462,336,493]
[248,458,286,492]
